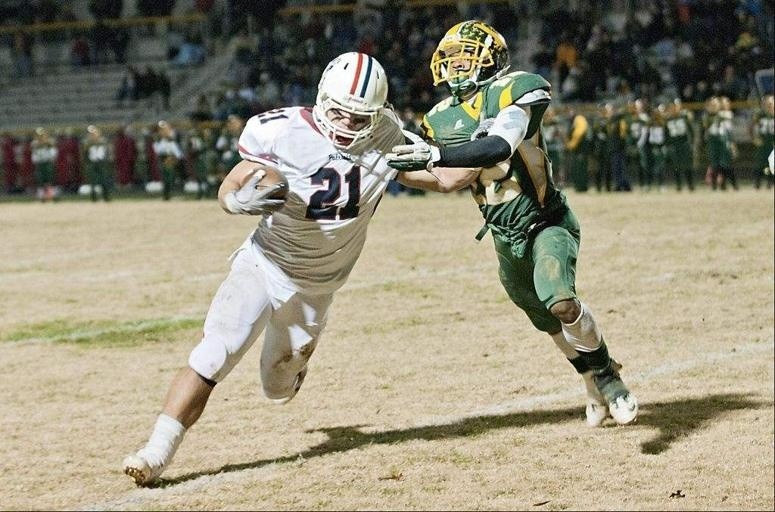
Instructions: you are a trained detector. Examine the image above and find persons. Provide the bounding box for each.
[121,51,497,486]
[326,19,640,427]
[2,0,775,200]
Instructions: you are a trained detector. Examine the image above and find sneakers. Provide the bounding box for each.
[121,443,169,485]
[581,357,640,429]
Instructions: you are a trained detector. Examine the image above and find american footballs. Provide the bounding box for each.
[240,167,290,202]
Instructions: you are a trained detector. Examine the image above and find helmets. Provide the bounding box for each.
[310,51,392,155]
[427,17,514,102]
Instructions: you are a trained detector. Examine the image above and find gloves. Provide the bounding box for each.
[386,127,436,176]
[468,116,499,144]
[221,168,287,216]
